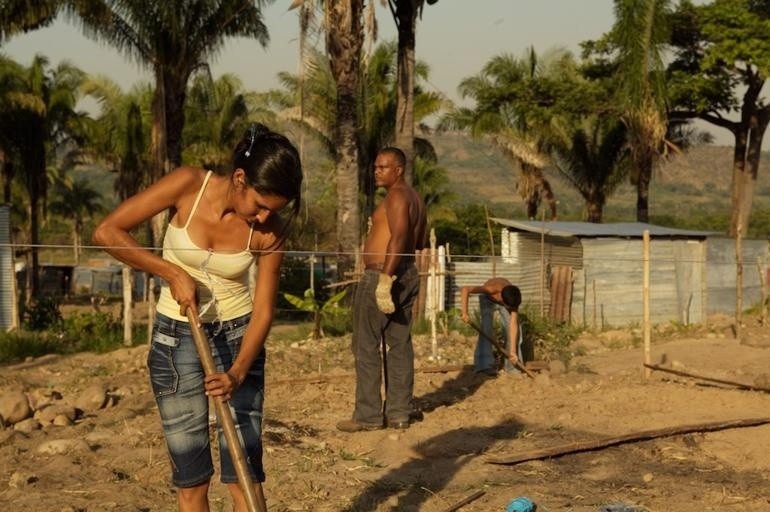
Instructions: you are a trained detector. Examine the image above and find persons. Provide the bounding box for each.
[335,146,428,432]
[457,277,527,372]
[93,122,303,511]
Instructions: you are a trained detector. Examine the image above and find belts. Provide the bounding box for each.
[364,261,416,271]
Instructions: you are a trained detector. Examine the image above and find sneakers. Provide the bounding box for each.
[335,417,411,433]
[481,366,498,378]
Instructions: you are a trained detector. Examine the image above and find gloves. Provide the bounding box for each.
[374,272,400,315]
